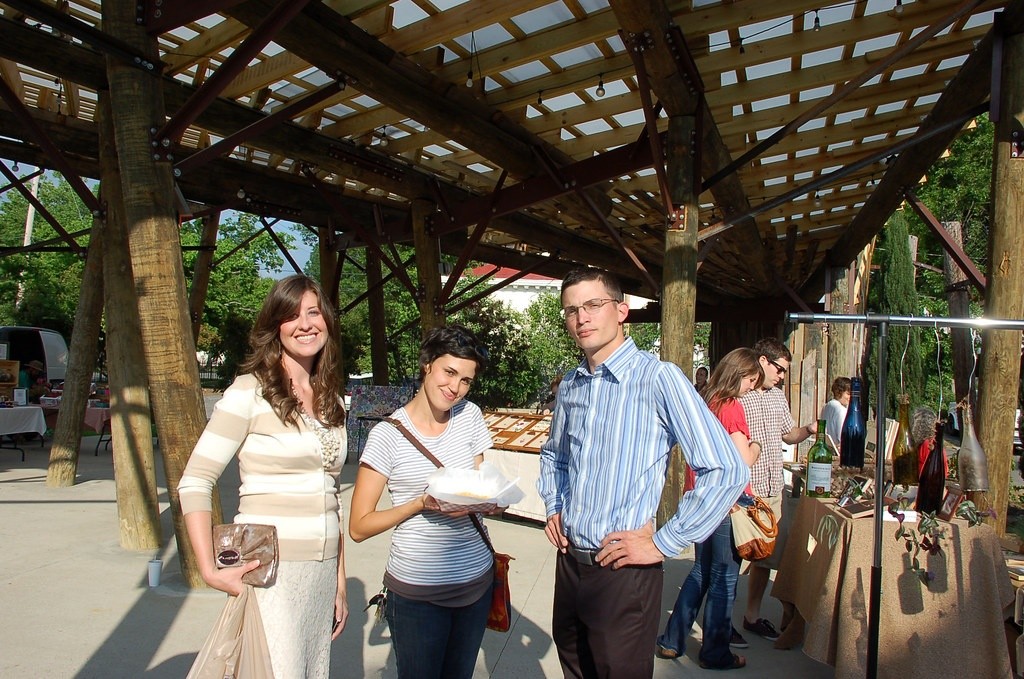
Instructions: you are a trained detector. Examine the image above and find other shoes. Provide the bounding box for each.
[700,653,746,669]
[656,643,676,658]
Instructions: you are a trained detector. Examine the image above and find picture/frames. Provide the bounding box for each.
[840,499,874,519]
[936,488,964,521]
[839,475,872,502]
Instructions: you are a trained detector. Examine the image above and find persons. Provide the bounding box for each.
[540,375,563,412]
[535,267,751,679]
[656,348,765,669]
[18,360,50,403]
[693,366,708,392]
[349,325,510,679]
[176,275,349,679]
[820,377,852,453]
[729,338,817,648]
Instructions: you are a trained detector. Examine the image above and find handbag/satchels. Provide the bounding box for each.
[186,584,273,678]
[487,551,515,632]
[212,523,279,588]
[730,496,778,561]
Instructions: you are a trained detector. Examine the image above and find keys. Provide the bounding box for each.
[361,590,389,627]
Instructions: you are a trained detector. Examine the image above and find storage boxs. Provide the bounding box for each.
[40,395,62,407]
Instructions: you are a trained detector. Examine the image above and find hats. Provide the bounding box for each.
[24,360,44,372]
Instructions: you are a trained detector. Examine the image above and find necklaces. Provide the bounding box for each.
[286,363,341,470]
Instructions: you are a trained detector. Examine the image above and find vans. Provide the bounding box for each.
[0,326,69,392]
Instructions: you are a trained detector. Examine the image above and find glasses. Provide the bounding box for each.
[559,298,620,321]
[768,358,789,375]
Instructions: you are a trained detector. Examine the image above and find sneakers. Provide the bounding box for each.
[728,624,748,648]
[743,616,781,641]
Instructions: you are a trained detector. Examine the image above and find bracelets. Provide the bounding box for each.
[749,441,762,457]
[806,423,812,435]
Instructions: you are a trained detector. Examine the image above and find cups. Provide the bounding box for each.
[148,560,163,586]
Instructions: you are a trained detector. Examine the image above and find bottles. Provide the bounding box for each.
[806,419,831,498]
[913,423,946,516]
[891,402,919,485]
[957,409,990,492]
[840,377,865,468]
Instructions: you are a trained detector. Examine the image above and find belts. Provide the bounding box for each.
[565,541,599,566]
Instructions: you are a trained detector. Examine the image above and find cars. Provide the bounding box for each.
[947,390,1023,447]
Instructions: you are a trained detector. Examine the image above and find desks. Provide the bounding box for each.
[769,487,1014,679]
[0,404,46,461]
[44,406,112,457]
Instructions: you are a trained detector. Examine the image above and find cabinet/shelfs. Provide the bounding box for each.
[0,360,20,403]
